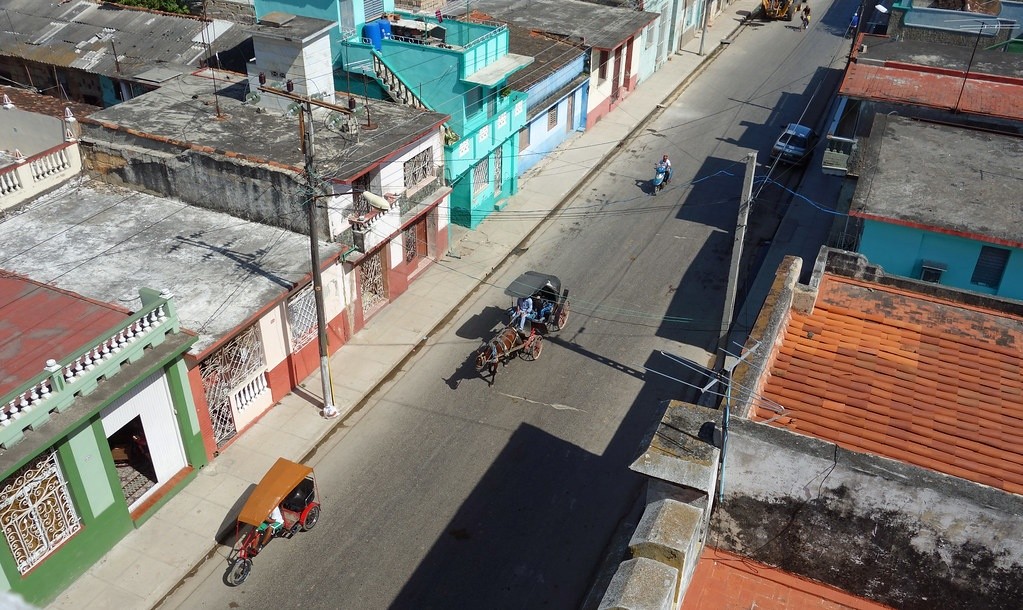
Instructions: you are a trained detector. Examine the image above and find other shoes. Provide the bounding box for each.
[519,328,523,331]
[806,25,808,28]
[256,544,262,552]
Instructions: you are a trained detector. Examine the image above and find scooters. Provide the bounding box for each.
[652,162,674,196]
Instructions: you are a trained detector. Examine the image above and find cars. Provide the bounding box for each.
[770,123,820,169]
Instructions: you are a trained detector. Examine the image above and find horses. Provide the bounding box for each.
[475,328,528,389]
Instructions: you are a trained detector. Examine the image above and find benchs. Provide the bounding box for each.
[510,298,554,324]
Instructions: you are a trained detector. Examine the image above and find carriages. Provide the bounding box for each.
[472,271,572,389]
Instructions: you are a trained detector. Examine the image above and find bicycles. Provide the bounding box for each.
[849,26,855,40]
[800,13,810,32]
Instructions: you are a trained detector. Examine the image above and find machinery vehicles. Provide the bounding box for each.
[761,0,802,22]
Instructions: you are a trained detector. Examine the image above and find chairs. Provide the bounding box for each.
[282,476,315,512]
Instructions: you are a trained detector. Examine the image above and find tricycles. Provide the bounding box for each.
[227,457,320,586]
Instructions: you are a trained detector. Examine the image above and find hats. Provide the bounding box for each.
[663,154,669,158]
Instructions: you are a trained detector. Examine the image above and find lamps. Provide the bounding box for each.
[875,4,892,16]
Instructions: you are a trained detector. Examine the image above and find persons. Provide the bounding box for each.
[250,506,284,553]
[801,5,810,28]
[511,298,553,331]
[849,13,859,34]
[133,433,146,453]
[654,155,671,182]
